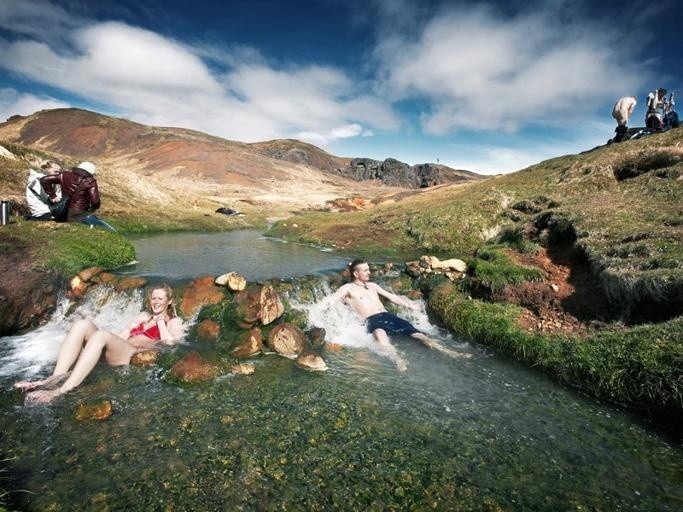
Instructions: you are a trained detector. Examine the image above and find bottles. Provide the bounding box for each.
[1,201,9,226]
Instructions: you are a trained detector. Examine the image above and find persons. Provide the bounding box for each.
[12,283,186,411]
[311,258,476,373]
[37,161,115,232]
[25,160,62,221]
[611,95,638,129]
[645,88,675,123]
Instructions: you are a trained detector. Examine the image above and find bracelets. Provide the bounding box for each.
[155,317,166,324]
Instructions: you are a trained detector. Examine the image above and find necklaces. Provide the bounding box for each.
[152,318,155,321]
[352,280,368,289]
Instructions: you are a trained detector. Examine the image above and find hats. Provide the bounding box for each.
[78,161,95,177]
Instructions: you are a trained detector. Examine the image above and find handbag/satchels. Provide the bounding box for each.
[47,194,70,222]
